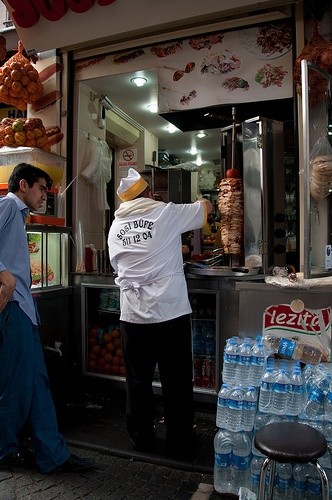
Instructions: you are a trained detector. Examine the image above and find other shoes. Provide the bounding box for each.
[133,437,165,452]
[166,439,210,458]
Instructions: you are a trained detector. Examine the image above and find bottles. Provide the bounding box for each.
[192,323,215,389]
[213,335,332,500]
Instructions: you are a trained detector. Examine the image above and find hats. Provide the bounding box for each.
[113,168,149,201]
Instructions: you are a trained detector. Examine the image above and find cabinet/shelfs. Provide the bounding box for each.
[81,283,220,399]
[140,169,194,252]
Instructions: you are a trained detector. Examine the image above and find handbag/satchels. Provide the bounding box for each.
[308,134,332,204]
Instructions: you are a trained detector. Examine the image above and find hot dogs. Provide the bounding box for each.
[33,63,64,145]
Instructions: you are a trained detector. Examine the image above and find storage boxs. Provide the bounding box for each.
[23,226,68,290]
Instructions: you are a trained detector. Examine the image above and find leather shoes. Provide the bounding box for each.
[0,448,39,469]
[48,454,98,476]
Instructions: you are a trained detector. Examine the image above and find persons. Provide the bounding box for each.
[0,163,94,471]
[108,168,213,455]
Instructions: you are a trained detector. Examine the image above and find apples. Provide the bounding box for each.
[0,117,51,152]
[88,326,124,376]
[0,58,44,110]
[294,40,332,105]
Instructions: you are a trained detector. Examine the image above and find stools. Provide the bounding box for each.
[254,422,330,500]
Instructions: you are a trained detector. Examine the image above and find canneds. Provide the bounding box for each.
[192,358,216,389]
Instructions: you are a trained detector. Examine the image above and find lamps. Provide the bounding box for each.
[130,77,147,87]
